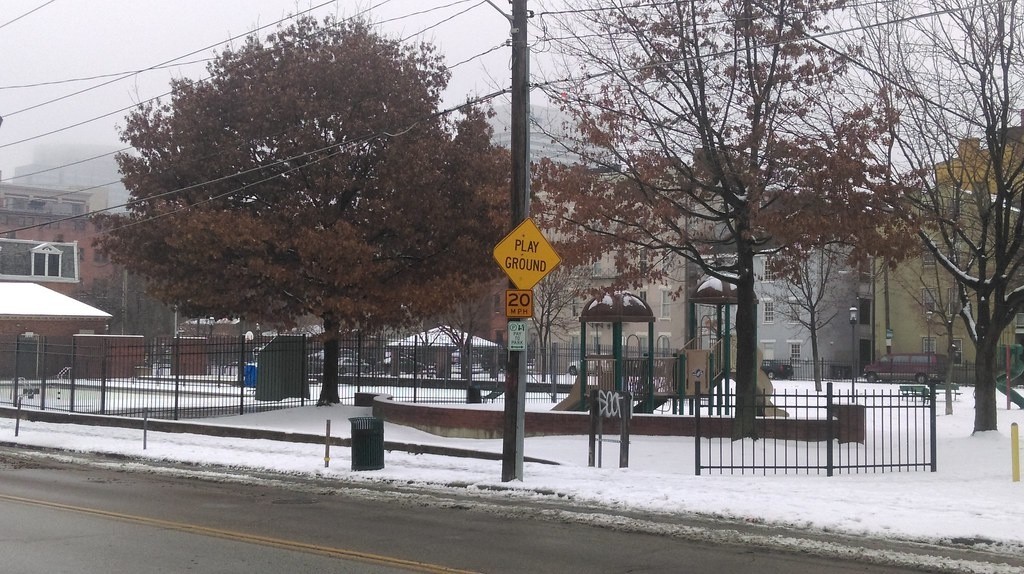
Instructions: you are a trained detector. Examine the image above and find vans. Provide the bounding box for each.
[863,352,948,384]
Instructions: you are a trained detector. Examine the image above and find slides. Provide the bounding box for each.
[996,344,1024,409]
[757,346,790,417]
[552,372,583,411]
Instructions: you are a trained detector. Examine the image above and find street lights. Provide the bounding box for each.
[208,317,215,375]
[255,323,261,359]
[849,306,858,404]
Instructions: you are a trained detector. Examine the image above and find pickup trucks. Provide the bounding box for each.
[761,365,794,379]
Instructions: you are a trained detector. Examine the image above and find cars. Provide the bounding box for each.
[338,359,372,375]
[567,352,614,376]
[452,349,481,363]
[318,350,356,361]
[375,357,425,374]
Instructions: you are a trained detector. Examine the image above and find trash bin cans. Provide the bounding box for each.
[466,385,482,403]
[348,416,385,471]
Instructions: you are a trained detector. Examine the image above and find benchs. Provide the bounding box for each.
[935,383,962,401]
[900,386,929,401]
[481,389,505,403]
